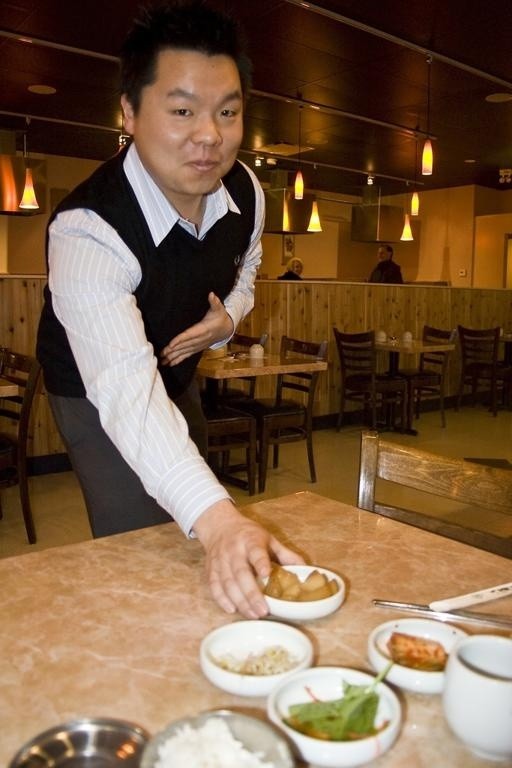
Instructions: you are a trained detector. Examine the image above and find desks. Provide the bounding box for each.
[370,332,456,370]
[196,345,329,381]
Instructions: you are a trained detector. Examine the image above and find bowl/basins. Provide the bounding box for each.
[198,619,315,698]
[266,665,404,768]
[377,331,386,343]
[368,617,469,695]
[8,718,149,768]
[402,331,412,342]
[139,709,294,767]
[249,344,264,355]
[254,564,347,621]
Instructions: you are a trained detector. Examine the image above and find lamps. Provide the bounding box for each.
[20,114,40,210]
[294,54,435,242]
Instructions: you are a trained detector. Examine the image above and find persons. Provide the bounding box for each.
[36,14,307,619]
[367,244,405,284]
[276,256,304,281]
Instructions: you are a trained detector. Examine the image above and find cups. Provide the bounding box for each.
[444,636,512,764]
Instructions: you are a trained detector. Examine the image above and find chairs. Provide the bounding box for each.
[0,349,40,544]
[235,335,330,489]
[334,327,410,438]
[456,326,512,419]
[386,325,458,430]
[221,332,268,399]
[188,377,258,497]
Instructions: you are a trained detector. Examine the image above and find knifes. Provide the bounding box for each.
[375,600,512,631]
